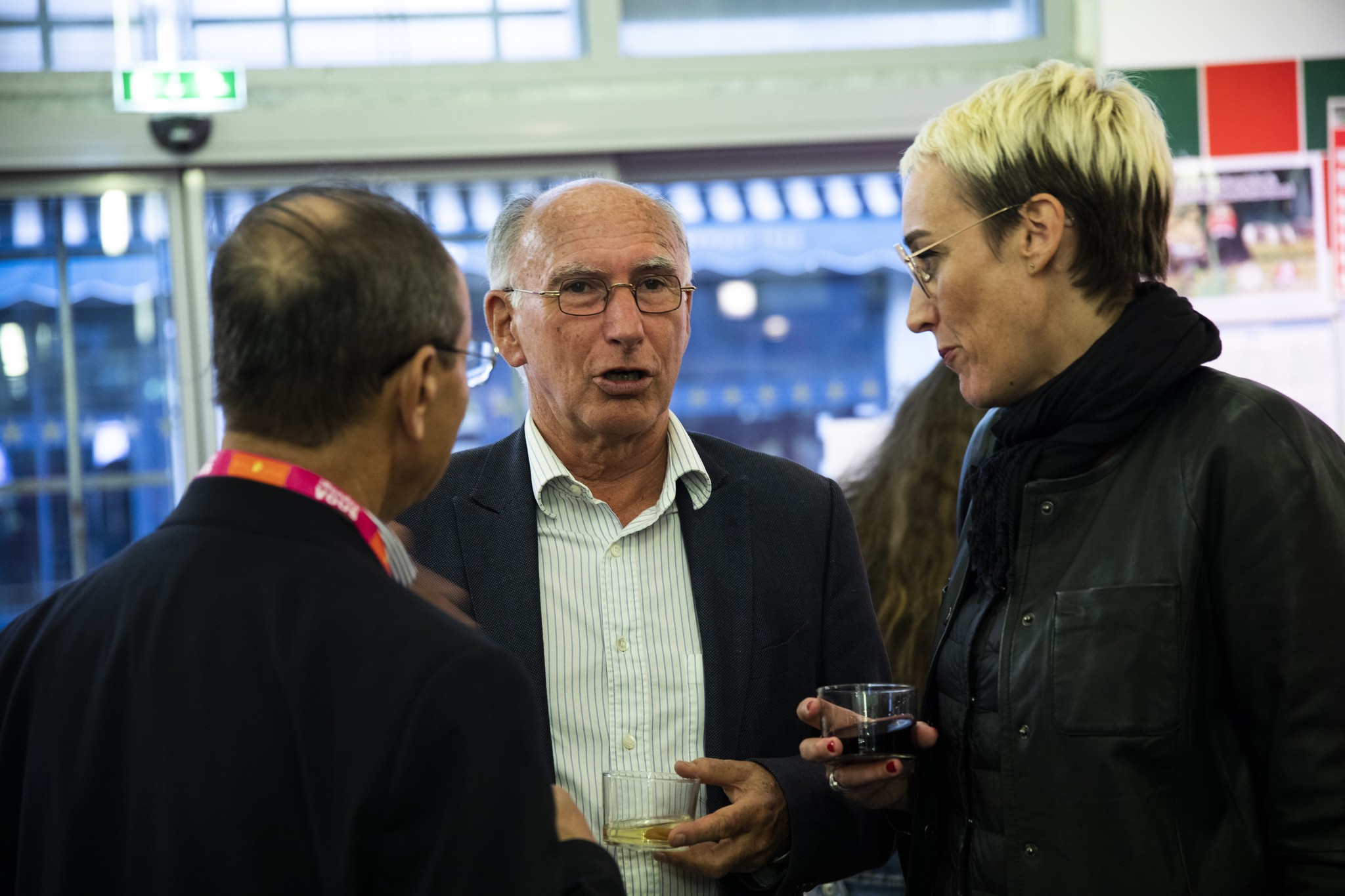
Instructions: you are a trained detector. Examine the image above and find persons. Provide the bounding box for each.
[1,186,627,894]
[389,168,915,894]
[840,358,995,685]
[788,59,1345,896]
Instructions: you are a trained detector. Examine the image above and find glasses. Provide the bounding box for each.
[894,198,1077,300]
[503,274,697,316]
[387,340,497,389]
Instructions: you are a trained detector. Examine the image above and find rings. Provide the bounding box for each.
[827,762,851,793]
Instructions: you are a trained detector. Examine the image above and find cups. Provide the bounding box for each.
[819,683,920,764]
[601,770,702,851]
